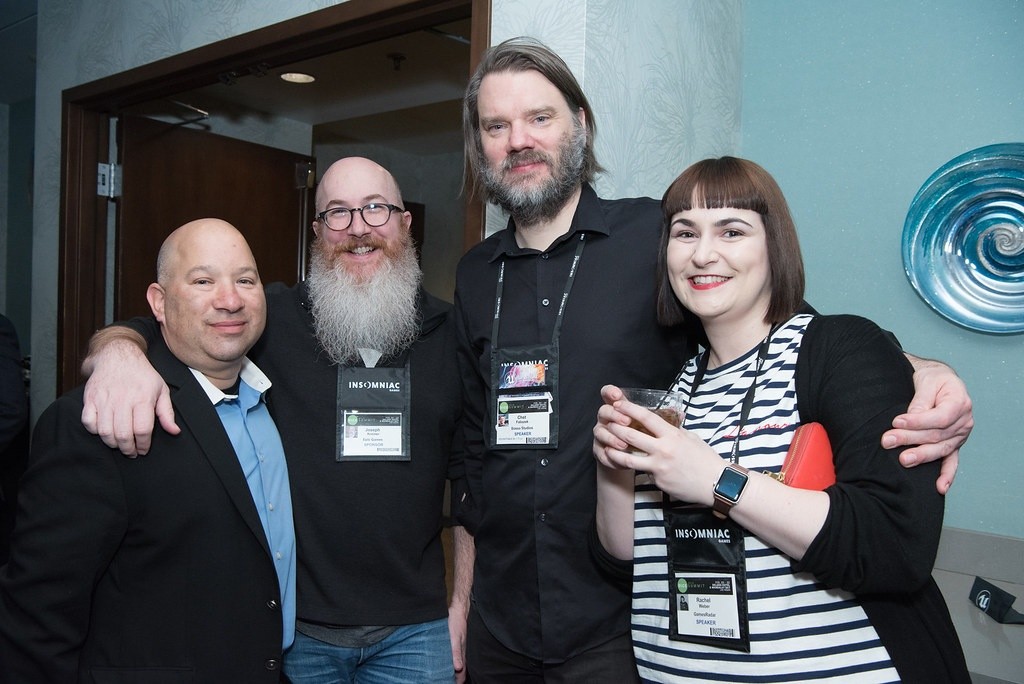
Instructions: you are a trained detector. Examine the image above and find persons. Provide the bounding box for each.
[19,218,300,684]
[348,426,356,438]
[80,156,475,684]
[453,33,975,684]
[680,596,688,610]
[592,155,972,684]
[499,416,508,427]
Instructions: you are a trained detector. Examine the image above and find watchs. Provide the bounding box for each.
[713,463,749,519]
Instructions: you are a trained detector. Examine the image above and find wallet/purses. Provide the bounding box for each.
[762,421,838,493]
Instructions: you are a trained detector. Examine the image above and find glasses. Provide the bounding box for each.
[316,202,405,232]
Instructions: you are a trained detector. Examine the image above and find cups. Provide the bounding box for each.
[621,387,684,455]
